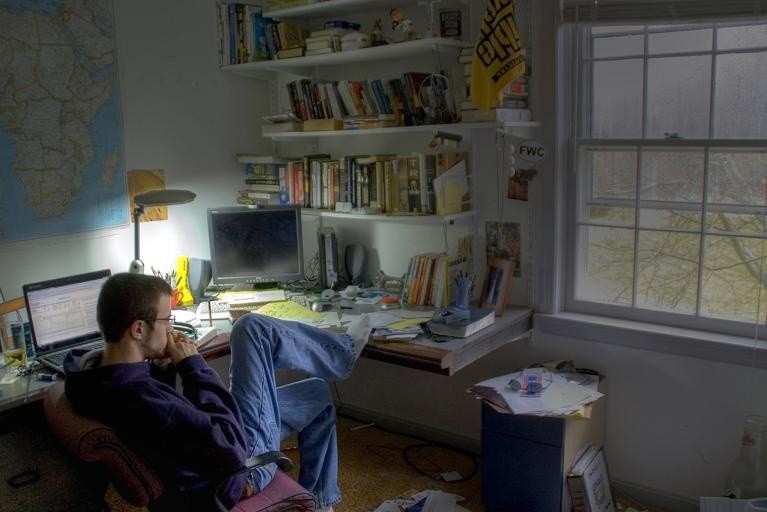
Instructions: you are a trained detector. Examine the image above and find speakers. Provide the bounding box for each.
[187,258,213,304]
[346,244,367,286]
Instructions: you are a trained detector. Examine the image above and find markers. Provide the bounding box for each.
[37,373,57,380]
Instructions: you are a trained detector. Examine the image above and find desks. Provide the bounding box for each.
[0,272,533,416]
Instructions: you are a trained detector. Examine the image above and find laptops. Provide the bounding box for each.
[23,268,113,376]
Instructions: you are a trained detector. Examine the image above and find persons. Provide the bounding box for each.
[63,273,375,512]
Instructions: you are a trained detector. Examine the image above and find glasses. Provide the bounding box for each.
[143,315,176,326]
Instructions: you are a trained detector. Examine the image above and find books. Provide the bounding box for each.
[566,443,614,512]
[401,253,496,338]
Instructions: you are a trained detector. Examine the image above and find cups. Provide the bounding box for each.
[0,322,27,373]
[170,288,183,307]
[456,281,474,309]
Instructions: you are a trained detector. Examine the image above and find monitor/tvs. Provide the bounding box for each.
[207,204,303,289]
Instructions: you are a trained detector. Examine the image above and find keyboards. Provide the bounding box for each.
[196,297,310,320]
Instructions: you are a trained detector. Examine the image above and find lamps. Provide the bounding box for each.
[128,189,196,274]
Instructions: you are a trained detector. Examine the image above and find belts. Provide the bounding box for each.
[244,450,295,474]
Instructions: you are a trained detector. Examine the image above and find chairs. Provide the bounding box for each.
[43,383,317,511]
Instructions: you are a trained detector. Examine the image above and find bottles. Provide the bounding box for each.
[724,414,767,501]
[279,185,288,206]
[317,227,338,289]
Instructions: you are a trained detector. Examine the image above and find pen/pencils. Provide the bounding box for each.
[341,306,353,309]
[151,266,182,289]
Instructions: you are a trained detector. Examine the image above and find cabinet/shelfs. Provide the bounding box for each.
[219,0,542,223]
[476,370,605,511]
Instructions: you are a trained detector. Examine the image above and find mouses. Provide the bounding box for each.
[312,301,333,312]
